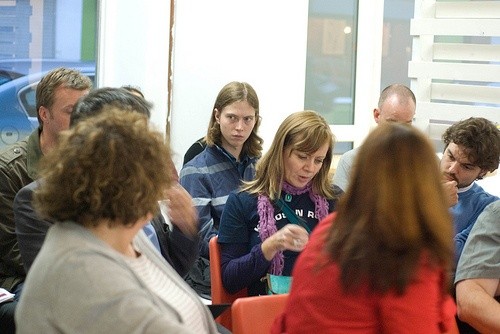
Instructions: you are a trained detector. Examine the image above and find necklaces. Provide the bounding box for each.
[284,192,293,203]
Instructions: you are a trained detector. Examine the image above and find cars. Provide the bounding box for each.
[0,60,95,131]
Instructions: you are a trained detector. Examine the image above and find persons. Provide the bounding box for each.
[182,136,209,166]
[0,67,93,302]
[216,109,348,296]
[177,81,265,243]
[331,84,417,197]
[13,87,236,333]
[0,300,18,334]
[269,121,460,333]
[452,198,500,333]
[12,102,219,334]
[119,85,146,98]
[438,115,500,283]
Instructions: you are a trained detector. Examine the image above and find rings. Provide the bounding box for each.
[291,237,298,247]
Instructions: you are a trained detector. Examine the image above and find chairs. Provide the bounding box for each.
[209,234,258,305]
[231,294,294,334]
